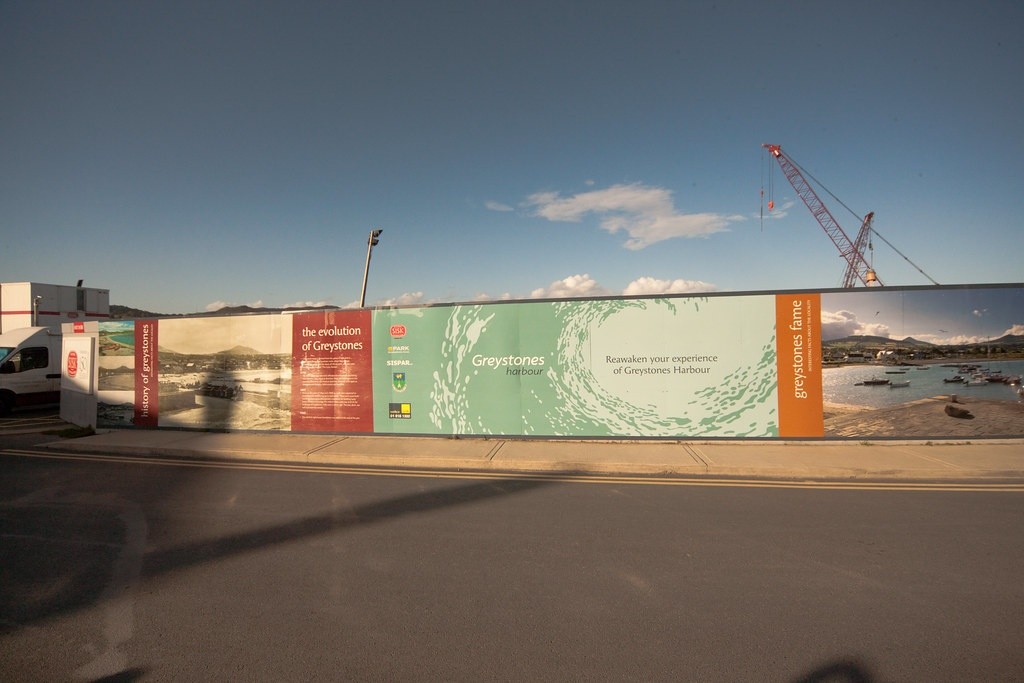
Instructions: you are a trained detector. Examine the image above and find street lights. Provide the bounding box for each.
[359,228,383,307]
[32,292,43,328]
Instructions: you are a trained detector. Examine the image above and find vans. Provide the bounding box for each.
[0,324,67,412]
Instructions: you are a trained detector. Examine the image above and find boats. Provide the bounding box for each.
[943,374,965,384]
[887,380,912,389]
[899,361,1024,395]
[944,404,971,419]
[863,377,890,386]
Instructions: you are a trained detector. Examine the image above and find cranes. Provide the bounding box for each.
[758,140,933,286]
